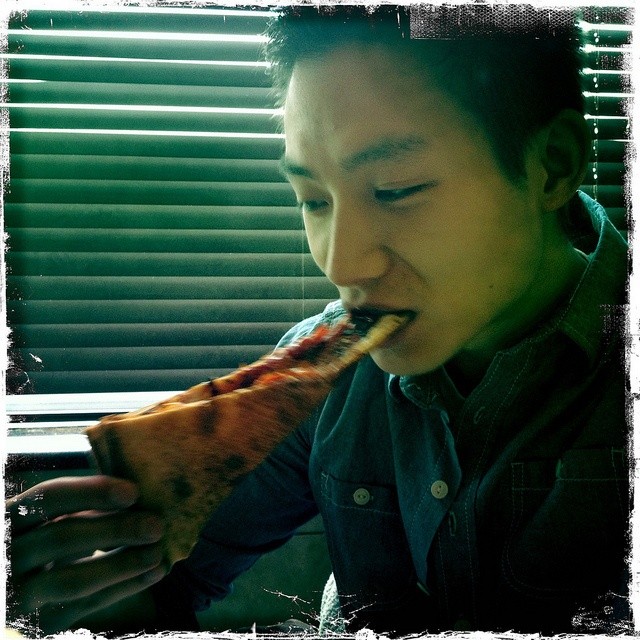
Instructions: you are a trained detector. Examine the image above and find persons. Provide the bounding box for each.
[4,8,627,630]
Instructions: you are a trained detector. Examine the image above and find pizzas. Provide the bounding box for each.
[83,312,414,566]
